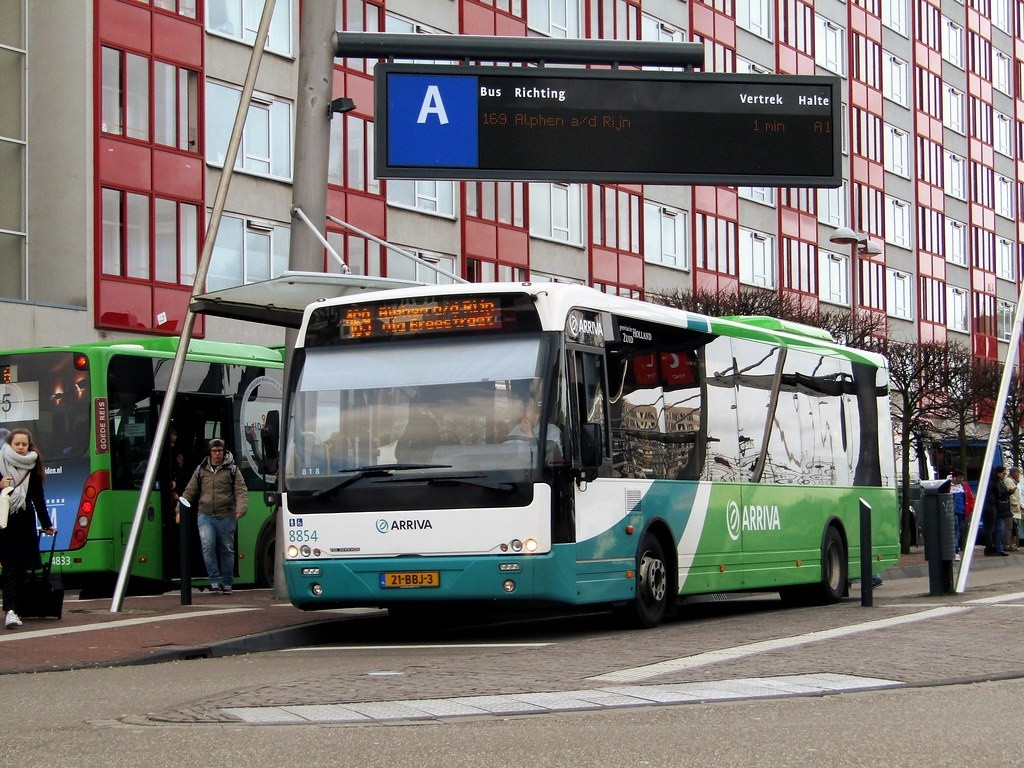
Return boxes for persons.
[159,428,193,562]
[982,466,1024,556]
[64,423,90,456]
[175,439,248,594]
[503,394,562,459]
[0,428,55,626]
[949,469,975,561]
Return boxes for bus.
[272,282,905,625]
[0,338,284,598]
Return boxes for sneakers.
[211,582,221,593]
[5,610,23,628]
[222,584,233,594]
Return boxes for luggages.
[19,529,65,619]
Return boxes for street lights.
[829,226,883,349]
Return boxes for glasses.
[210,449,223,453]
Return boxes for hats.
[209,439,224,448]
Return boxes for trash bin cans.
[918,476,959,600]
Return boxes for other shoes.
[955,553,961,561]
[983,548,1009,556]
[1003,544,1020,551]
